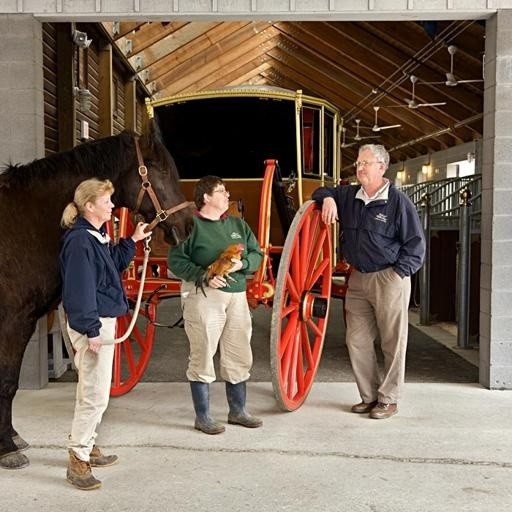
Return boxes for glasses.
[353,161,381,167]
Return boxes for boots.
[190,381,225,434]
[226,381,263,428]
[67,445,118,490]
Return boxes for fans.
[340,46,483,150]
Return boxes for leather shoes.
[352,399,397,419]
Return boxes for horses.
[0,117,193,470]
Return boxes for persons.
[310,143,429,421]
[56,177,154,489]
[165,175,265,437]
